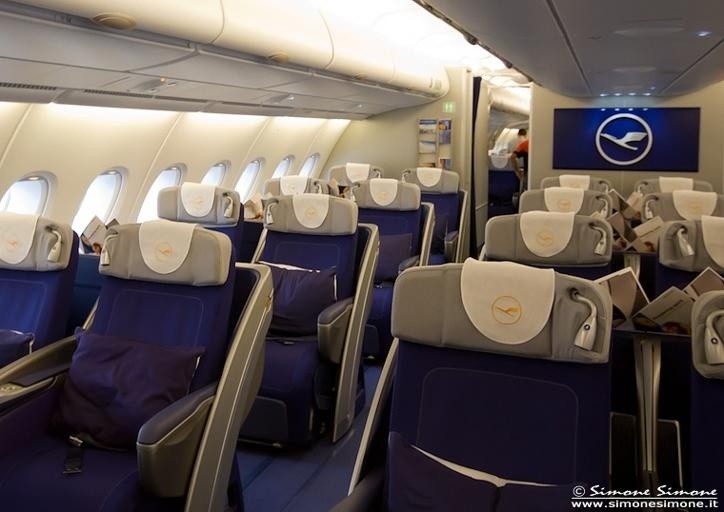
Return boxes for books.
[594,187,724,332]
[105,217,120,230]
[79,216,107,254]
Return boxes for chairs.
[0,161,723,507]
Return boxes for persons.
[511,139,529,191]
[510,128,528,190]
